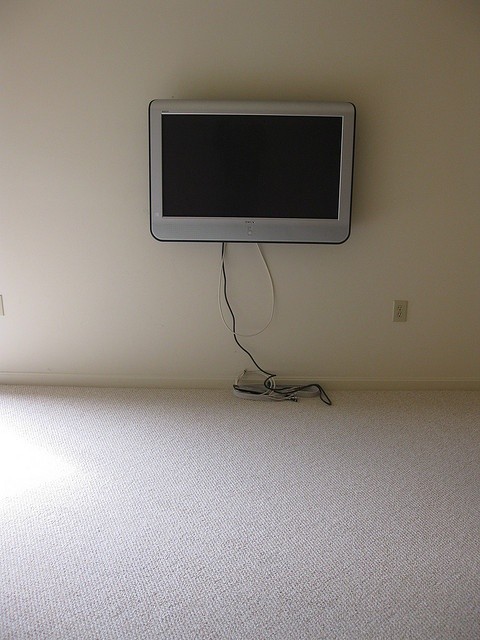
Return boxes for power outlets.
[393,299,408,322]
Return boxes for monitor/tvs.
[146,97,356,247]
